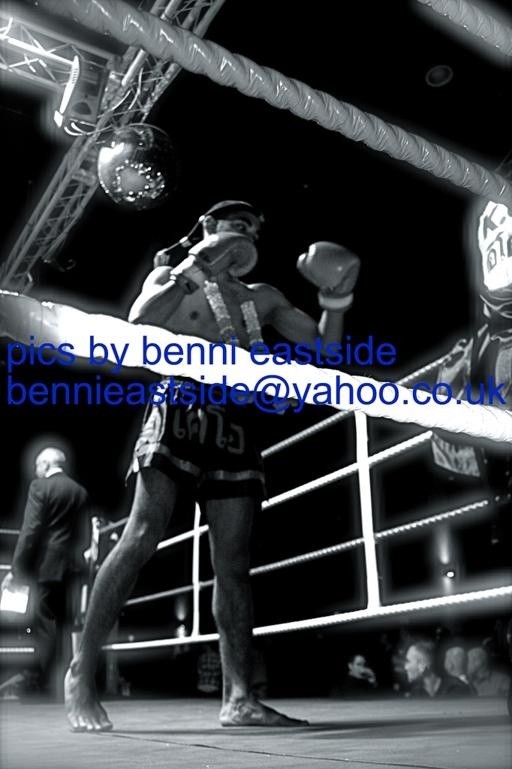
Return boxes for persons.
[3,447,88,703]
[63,198,363,735]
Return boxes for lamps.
[60,54,175,212]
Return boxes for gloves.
[296,242,360,311]
[171,232,260,294]
